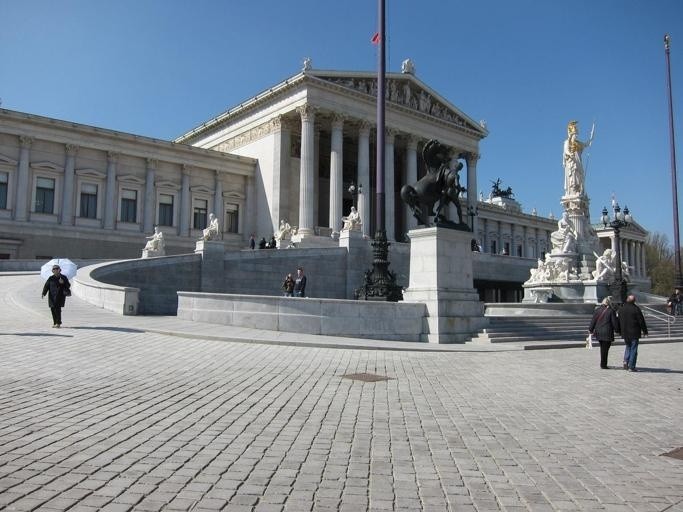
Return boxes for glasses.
[53,267,59,270]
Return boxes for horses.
[400,139,451,228]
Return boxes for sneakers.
[623,361,628,370]
[628,368,638,372]
[52,324,60,328]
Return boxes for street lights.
[600,192,633,307]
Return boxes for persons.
[592,248,631,282]
[342,206,361,231]
[283,273,295,297]
[588,295,620,369]
[293,267,307,297]
[269,236,276,248]
[258,237,266,249]
[667,292,683,315]
[526,254,578,280]
[199,213,219,240]
[275,220,291,240]
[550,210,575,253]
[142,225,164,250]
[248,235,254,249]
[433,162,468,224]
[562,119,594,197]
[41,264,71,328]
[617,295,648,371]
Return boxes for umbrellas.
[41,258,77,280]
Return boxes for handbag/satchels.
[63,288,72,296]
